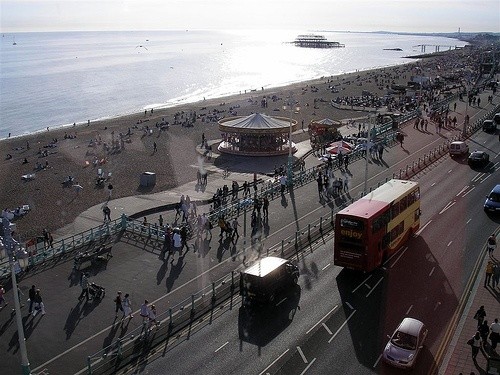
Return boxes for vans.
[449,141,469,159]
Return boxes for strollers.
[88,282,105,296]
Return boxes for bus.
[333,178,422,274]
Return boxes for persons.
[77,272,92,301]
[232,217,242,237]
[491,264,500,289]
[103,206,111,222]
[148,305,160,329]
[42,228,49,248]
[172,228,183,258]
[174,202,181,217]
[246,181,251,195]
[399,134,404,147]
[343,176,350,193]
[470,330,483,361]
[186,194,190,207]
[157,214,163,226]
[242,179,247,195]
[344,154,350,169]
[77,242,107,261]
[324,175,330,189]
[341,178,344,190]
[17,243,29,273]
[478,320,490,349]
[333,175,339,193]
[412,116,420,130]
[16,284,25,309]
[483,260,493,289]
[338,151,343,167]
[278,173,288,197]
[231,182,236,200]
[196,168,202,184]
[372,141,377,160]
[80,272,85,289]
[377,139,389,160]
[195,214,203,237]
[473,305,487,330]
[164,228,173,254]
[180,193,185,204]
[113,290,125,318]
[489,319,500,350]
[218,214,227,238]
[142,216,148,226]
[257,196,263,217]
[107,182,113,197]
[200,213,209,235]
[47,232,54,249]
[316,173,323,192]
[361,140,367,157]
[338,178,342,192]
[203,171,209,185]
[208,145,213,156]
[263,195,270,217]
[140,299,149,325]
[202,218,214,239]
[180,226,189,253]
[250,193,258,217]
[327,153,333,170]
[28,285,36,314]
[235,180,239,199]
[487,233,498,258]
[424,119,428,131]
[369,139,373,158]
[32,289,46,318]
[122,294,134,321]
[226,221,232,236]
[420,117,425,130]
[91,280,105,292]
[0,285,8,309]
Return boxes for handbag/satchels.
[466,338,474,345]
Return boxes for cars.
[467,149,489,166]
[483,184,500,214]
[382,317,429,370]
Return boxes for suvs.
[483,119,497,131]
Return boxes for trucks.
[239,256,300,311]
